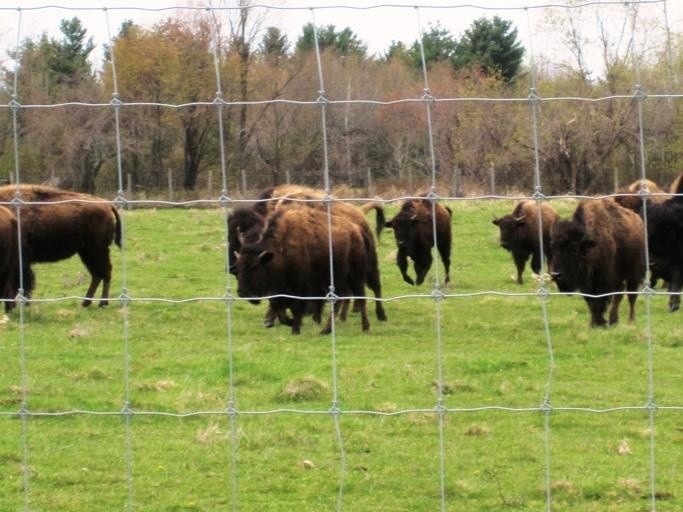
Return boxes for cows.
[546,170,683,328]
[0,183,121,324]
[226,181,386,336]
[386,191,454,287]
[490,200,561,285]
